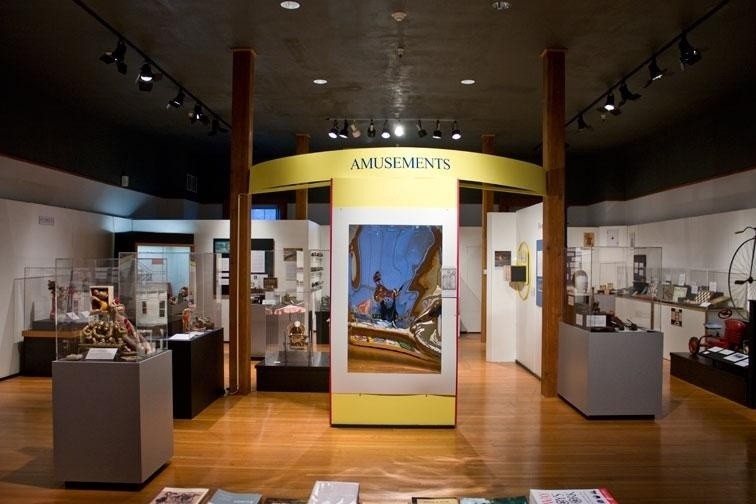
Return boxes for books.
[412,497,460,504]
[308,480,360,504]
[528,488,618,504]
[205,488,263,504]
[150,486,212,504]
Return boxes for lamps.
[326,118,461,140]
[565,2,729,131]
[73,0,231,134]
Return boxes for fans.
[728,237,756,321]
